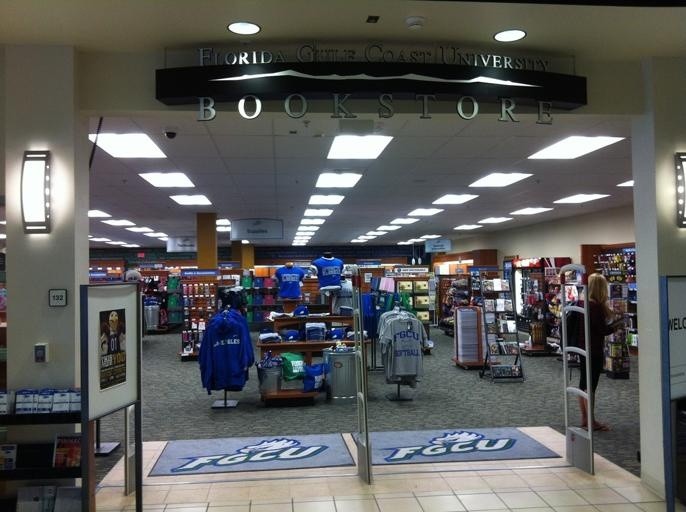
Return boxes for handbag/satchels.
[558,301,579,354]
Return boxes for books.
[482,278,522,377]
[604,285,630,373]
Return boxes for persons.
[310,250,346,293]
[121,262,143,281]
[271,259,308,297]
[100,311,126,355]
[577,272,624,430]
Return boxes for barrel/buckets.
[322,346,361,406]
[144,305,160,329]
[257,366,280,393]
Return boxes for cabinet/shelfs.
[544,276,637,380]
[177,279,237,363]
[436,273,502,338]
[142,269,295,337]
[321,266,430,355]
[0,411,82,512]
[253,315,356,402]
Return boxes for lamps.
[674,152,686,228]
[494,28,526,43]
[226,22,262,36]
[19,150,50,234]
